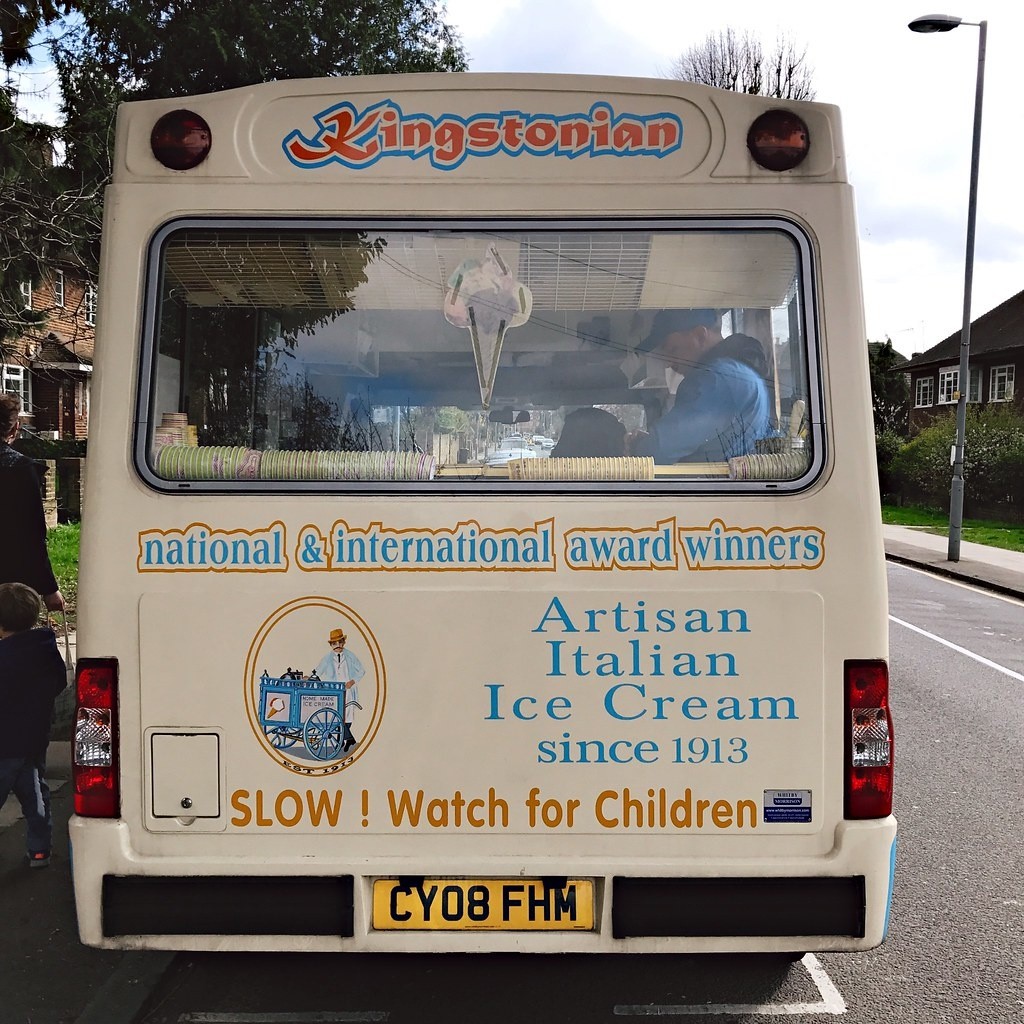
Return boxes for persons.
[0,392,65,871]
[552,309,771,465]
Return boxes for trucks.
[66,78,896,961]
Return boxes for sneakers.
[28,849,53,869]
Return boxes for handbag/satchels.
[45,609,77,742]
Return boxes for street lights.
[911,13,988,563]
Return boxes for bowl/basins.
[509,456,655,479]
[153,412,436,480]
[728,452,808,481]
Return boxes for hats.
[635,310,719,356]
